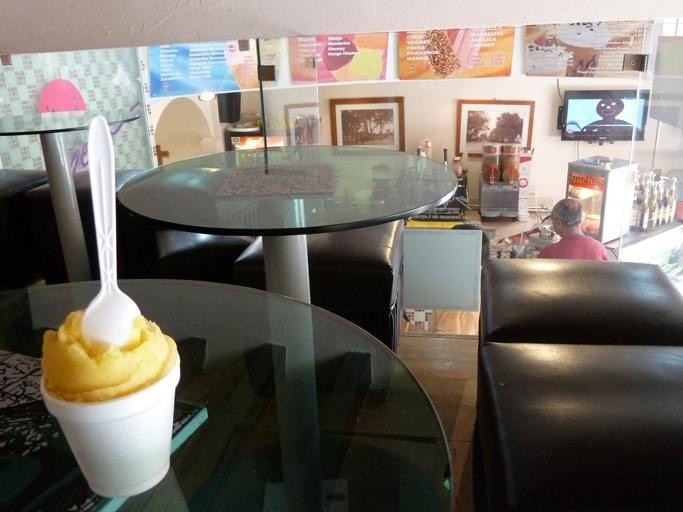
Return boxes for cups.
[38,347,183,500]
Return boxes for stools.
[0,167,405,357]
[482,258,683,346]
[482,341,683,512]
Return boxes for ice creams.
[526,37,568,72]
[560,22,607,46]
[41,307,177,404]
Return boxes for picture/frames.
[455,101,533,163]
[328,96,405,154]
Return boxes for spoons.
[78,113,144,349]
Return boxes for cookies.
[422,30,462,79]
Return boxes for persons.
[536,198,608,261]
[423,224,491,337]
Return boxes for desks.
[0,278,457,510]
[117,144,458,304]
[1,110,143,283]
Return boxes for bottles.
[628,171,678,234]
